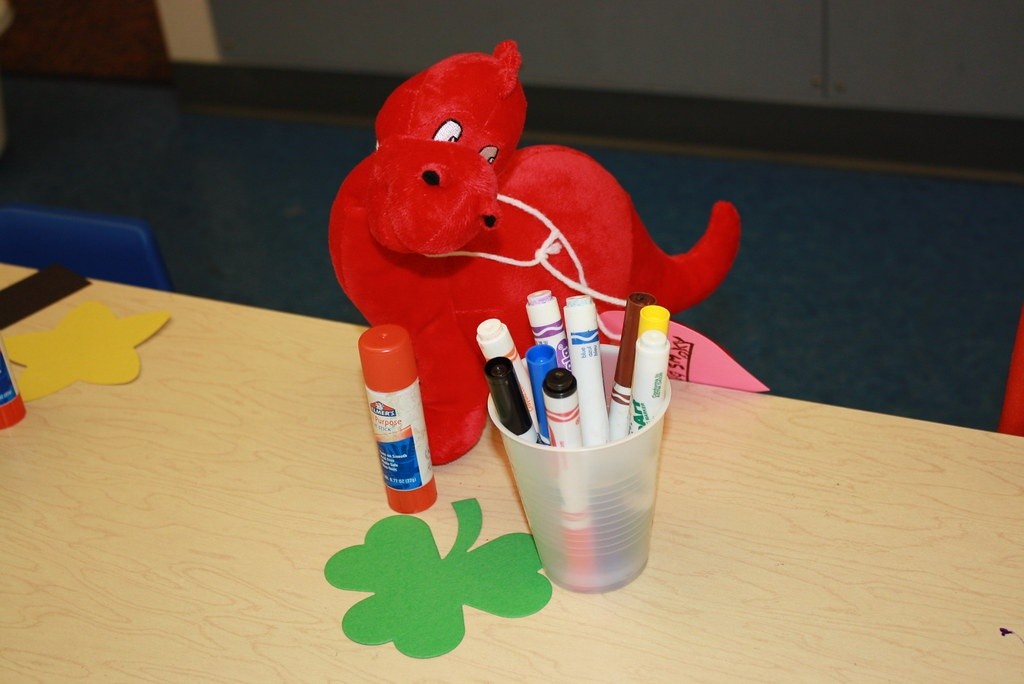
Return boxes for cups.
[487,344,671,593]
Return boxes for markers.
[476,289,671,445]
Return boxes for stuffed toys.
[328,38,744,466]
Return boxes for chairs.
[0,205,174,292]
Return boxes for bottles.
[358,323,438,514]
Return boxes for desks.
[0,261,1024,684]
